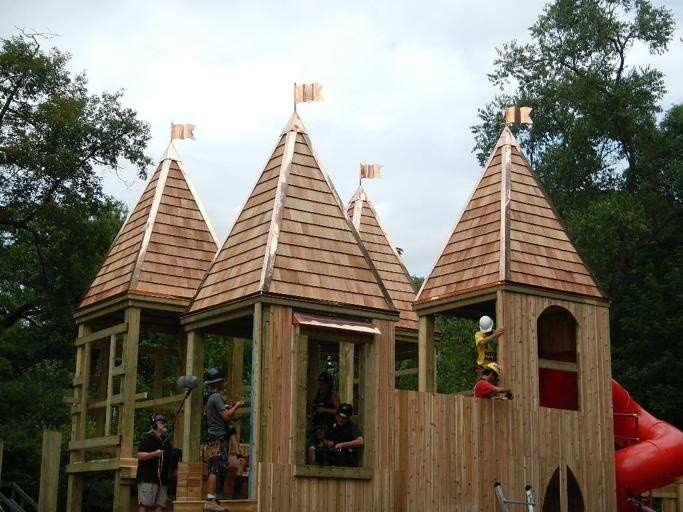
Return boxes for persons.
[304,372,364,468]
[225,423,247,500]
[202,367,245,512]
[475,315,505,370]
[473,362,512,398]
[138,413,173,512]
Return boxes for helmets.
[337,402,353,419]
[204,368,224,384]
[479,315,493,333]
[484,362,500,374]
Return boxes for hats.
[154,415,167,421]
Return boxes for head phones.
[152,414,158,430]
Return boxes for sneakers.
[203,500,229,512]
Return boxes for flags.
[294,84,322,102]
[507,107,533,124]
[172,124,196,140]
[361,165,381,179]
